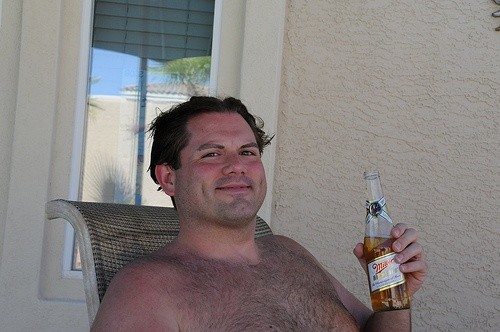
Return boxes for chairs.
[46,198,277,332]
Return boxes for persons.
[87,95,428,331]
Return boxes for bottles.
[362,170,410,312]
